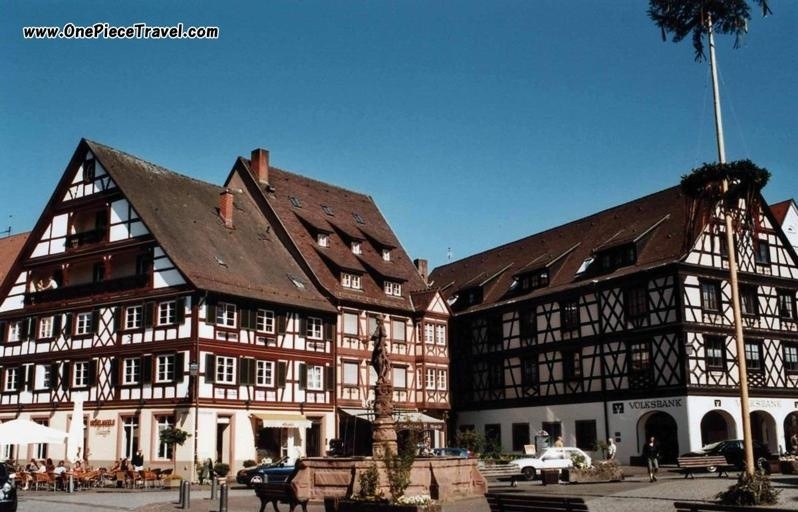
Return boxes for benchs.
[672,455,735,480]
[475,462,592,512]
[255,482,310,511]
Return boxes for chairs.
[9,467,174,493]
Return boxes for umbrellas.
[0,420,65,467]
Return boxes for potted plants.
[214,463,230,485]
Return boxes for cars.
[504,447,592,480]
[678,439,772,473]
[433,447,479,459]
[237,456,300,488]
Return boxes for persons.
[37,275,58,291]
[554,436,564,447]
[196,458,220,485]
[643,436,660,482]
[3,446,144,491]
[607,438,617,460]
